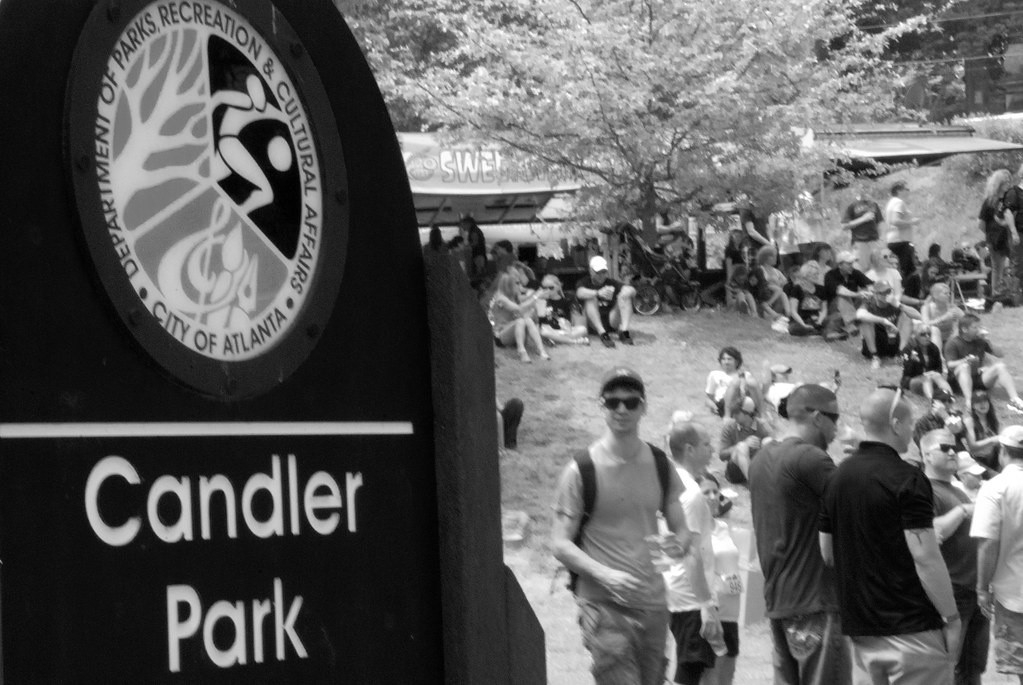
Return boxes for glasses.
[540,285,555,290]
[928,443,958,454]
[805,406,840,423]
[882,255,893,259]
[600,397,644,411]
[920,331,931,337]
[940,398,954,404]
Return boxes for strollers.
[614,219,704,318]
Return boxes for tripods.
[934,267,970,314]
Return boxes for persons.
[705,346,1023,684]
[423,215,590,360]
[723,162,1022,415]
[659,409,741,685]
[552,365,690,685]
[576,255,639,348]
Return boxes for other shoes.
[617,330,633,345]
[600,333,614,348]
[517,349,531,364]
[826,331,849,341]
[574,337,590,345]
[871,357,881,371]
[1006,396,1023,413]
[536,349,550,361]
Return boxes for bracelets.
[532,295,537,300]
[962,504,970,515]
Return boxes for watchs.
[942,614,963,625]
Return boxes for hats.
[971,389,990,404]
[770,364,792,376]
[956,460,986,475]
[836,251,859,263]
[931,388,953,400]
[599,365,644,396]
[874,279,894,293]
[589,255,608,272]
[999,424,1023,448]
